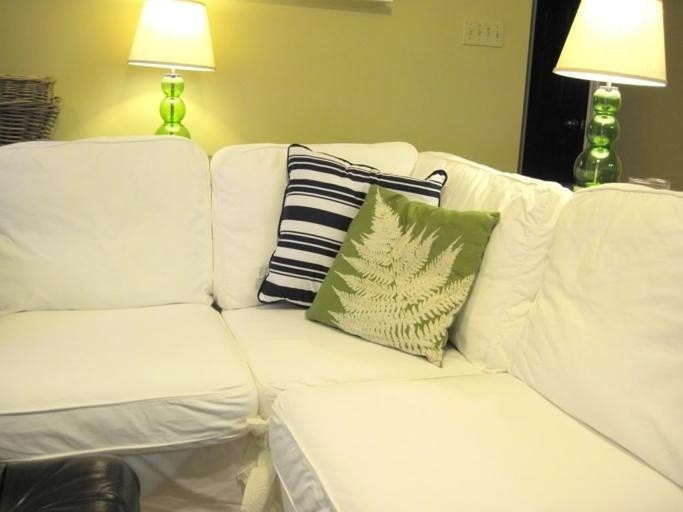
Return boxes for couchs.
[3,140,681,510]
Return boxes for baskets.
[0,72,63,145]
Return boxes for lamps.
[550,0,668,188]
[127,1,216,139]
